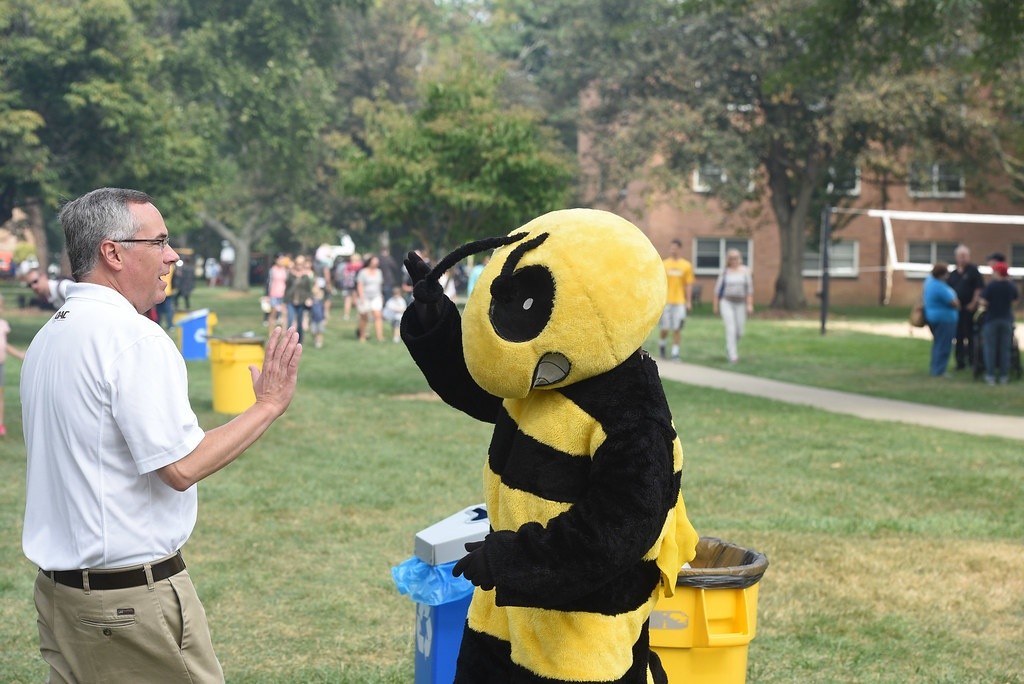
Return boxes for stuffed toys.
[400,207,699,684]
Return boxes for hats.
[990,262,1009,278]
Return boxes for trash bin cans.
[203,335,265,413]
[172,310,218,353]
[646,537,769,684]
[416,503,488,684]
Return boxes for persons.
[0,296,26,435]
[260,251,332,348]
[713,250,753,360]
[467,255,490,294]
[923,262,960,376]
[145,258,195,329]
[438,272,455,299]
[22,187,302,684]
[206,240,236,287]
[25,268,74,309]
[336,246,425,343]
[660,239,694,356]
[948,245,1019,385]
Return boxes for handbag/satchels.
[911,305,925,329]
[717,267,727,300]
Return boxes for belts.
[41,552,185,590]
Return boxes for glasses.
[113,235,170,249]
[26,275,41,288]
